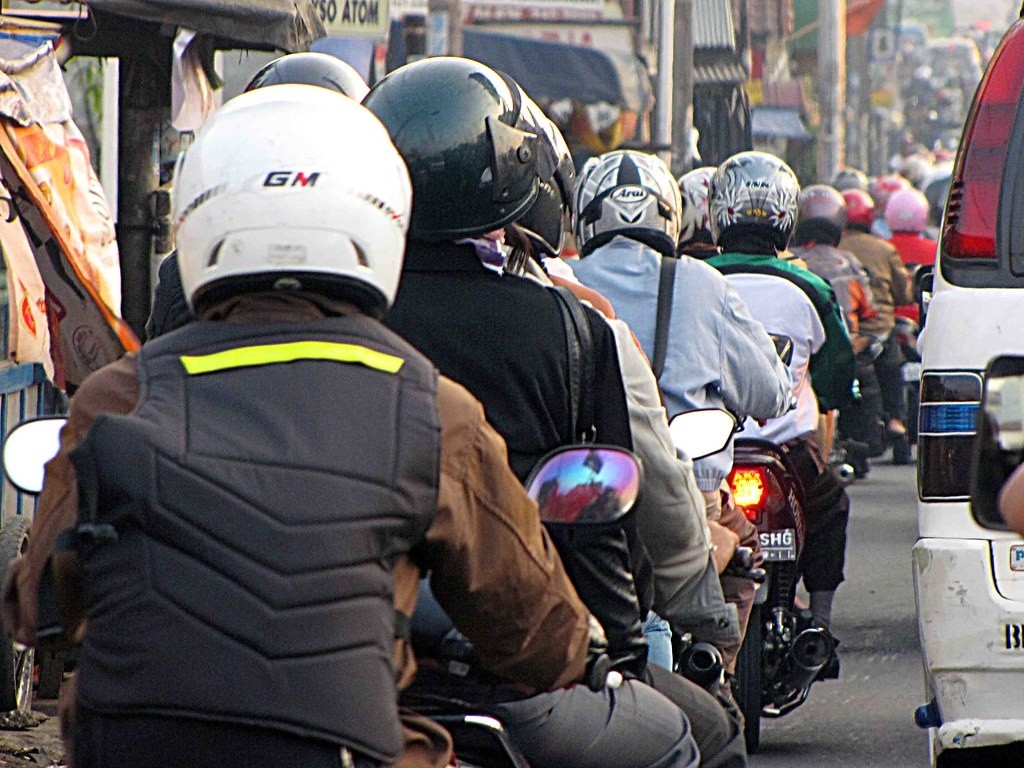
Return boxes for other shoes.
[713,679,746,728]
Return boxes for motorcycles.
[1,260,933,767]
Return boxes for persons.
[0,85,591,768]
[142,53,1024,768]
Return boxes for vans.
[902,7,1023,768]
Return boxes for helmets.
[793,184,847,246]
[841,188,875,233]
[707,152,800,250]
[676,167,722,248]
[243,51,371,104]
[513,120,577,256]
[360,57,559,243]
[168,83,412,313]
[833,168,867,192]
[884,188,927,235]
[574,148,683,258]
